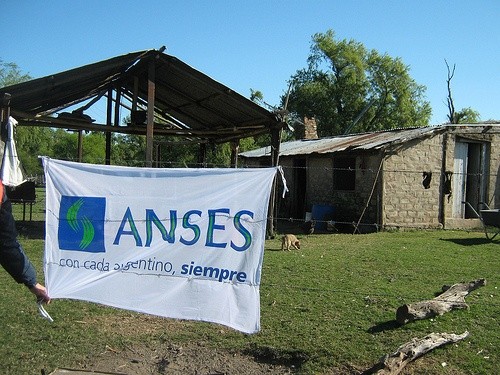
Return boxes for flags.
[37,154,290,335]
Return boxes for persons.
[0,179,51,306]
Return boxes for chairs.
[6,180,36,224]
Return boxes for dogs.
[278,234,301,252]
